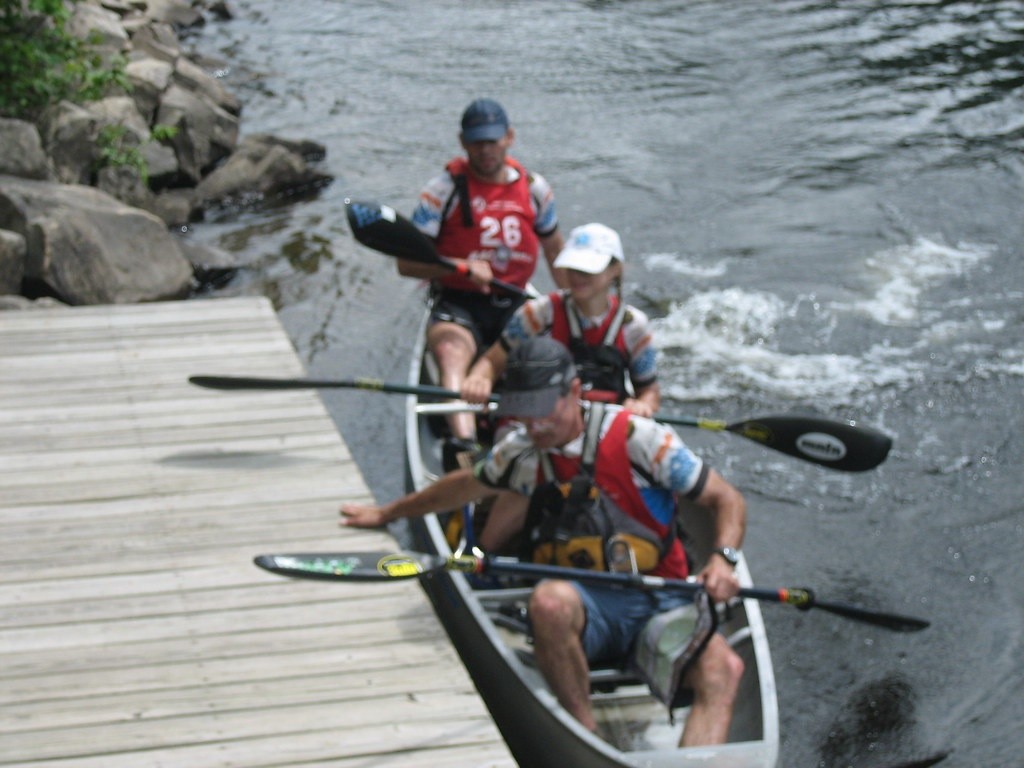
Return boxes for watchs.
[714,546,739,566]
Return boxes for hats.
[461,97,509,140]
[553,223,625,274]
[492,336,574,417]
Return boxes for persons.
[339,336,748,748]
[395,97,570,473]
[461,221,660,575]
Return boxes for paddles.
[253,550,934,634]
[345,201,537,299]
[188,356,898,475]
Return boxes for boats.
[402,276,784,767]
